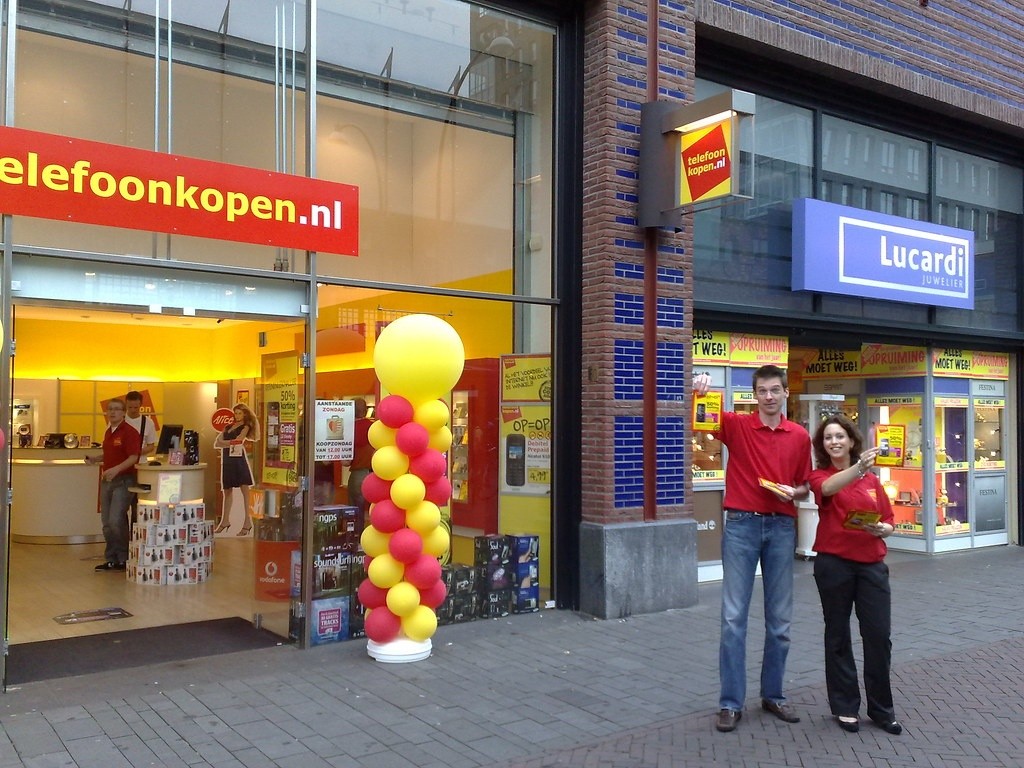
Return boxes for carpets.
[5,617,291,686]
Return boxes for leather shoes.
[717,706,748,731]
[761,698,800,722]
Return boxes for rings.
[866,458,869,462]
[868,453,871,456]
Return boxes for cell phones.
[506,434,526,486]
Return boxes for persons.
[693,366,903,736]
[84,391,159,572]
[214,403,260,536]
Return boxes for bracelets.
[855,459,865,480]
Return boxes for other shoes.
[95,561,126,572]
[833,712,865,733]
[873,713,902,735]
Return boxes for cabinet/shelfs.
[890,467,964,534]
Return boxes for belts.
[724,508,797,518]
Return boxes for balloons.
[0,318,5,452]
[357,313,465,644]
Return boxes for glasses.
[108,406,127,415]
[758,385,784,396]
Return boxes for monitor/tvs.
[156,424,183,454]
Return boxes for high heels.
[237,523,253,538]
[213,521,233,534]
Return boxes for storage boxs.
[290,505,539,645]
[126,503,215,586]
